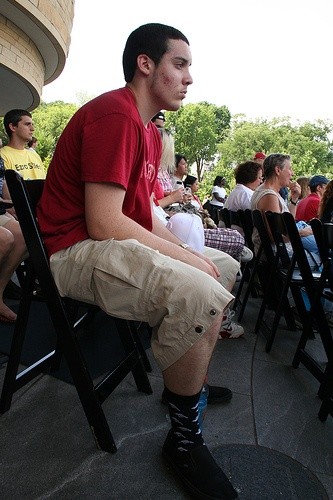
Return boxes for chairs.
[0,169,333,457]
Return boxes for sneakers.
[217,308,244,339]
[162,382,233,405]
[161,428,239,500]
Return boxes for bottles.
[175,181,192,203]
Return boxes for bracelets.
[178,242,189,250]
[290,198,297,207]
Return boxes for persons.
[0,109,46,322]
[151,111,333,339]
[37,23,240,500]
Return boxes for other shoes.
[295,318,319,332]
[324,311,333,328]
[241,245,253,262]
[235,268,242,282]
[0,303,17,323]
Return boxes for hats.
[310,175,329,187]
[152,112,165,120]
[255,153,266,159]
